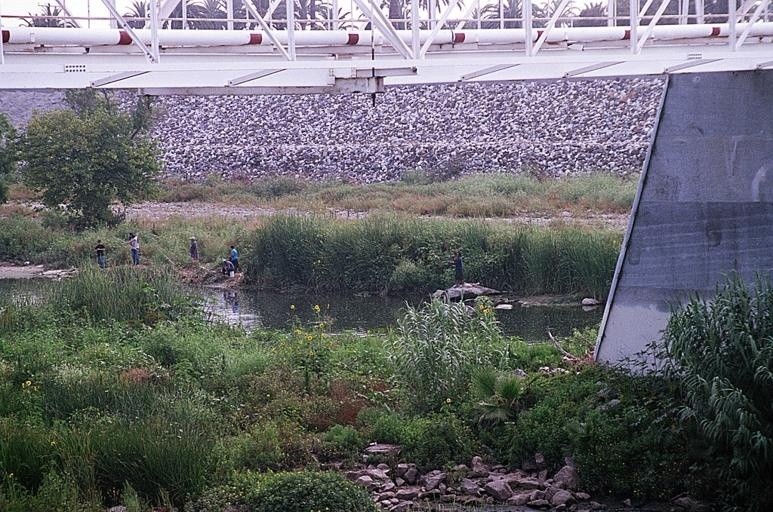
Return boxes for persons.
[184,236,199,261]
[120,232,139,265]
[228,246,238,273]
[93,239,106,268]
[448,250,464,288]
[221,259,234,277]
